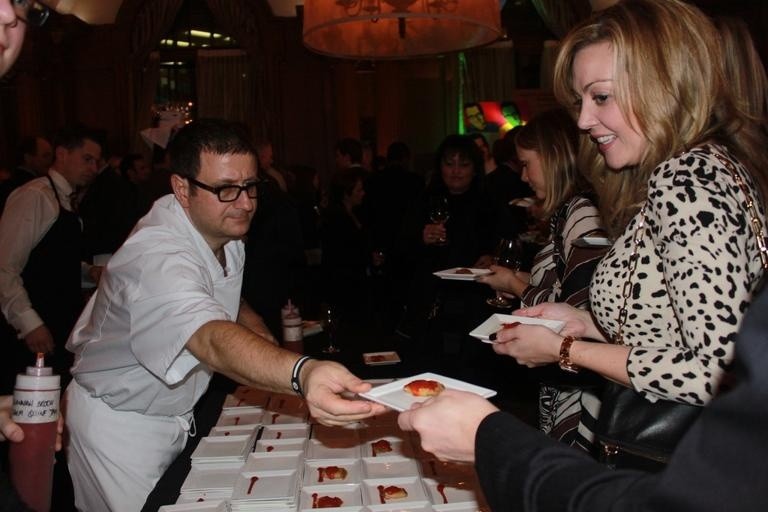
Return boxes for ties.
[70,192,80,215]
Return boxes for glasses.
[192,177,265,203]
[10,1,53,29]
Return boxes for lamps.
[301,2,502,63]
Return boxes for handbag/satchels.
[591,378,706,474]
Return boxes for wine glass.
[486,238,523,310]
[427,195,451,247]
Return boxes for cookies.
[321,468,347,479]
[316,496,344,509]
[372,440,392,457]
[404,380,446,396]
[384,487,407,500]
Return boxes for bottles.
[7,350,63,512]
[279,296,305,356]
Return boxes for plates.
[359,371,499,418]
[468,312,567,346]
[360,350,401,368]
[157,407,474,512]
[429,265,494,282]
